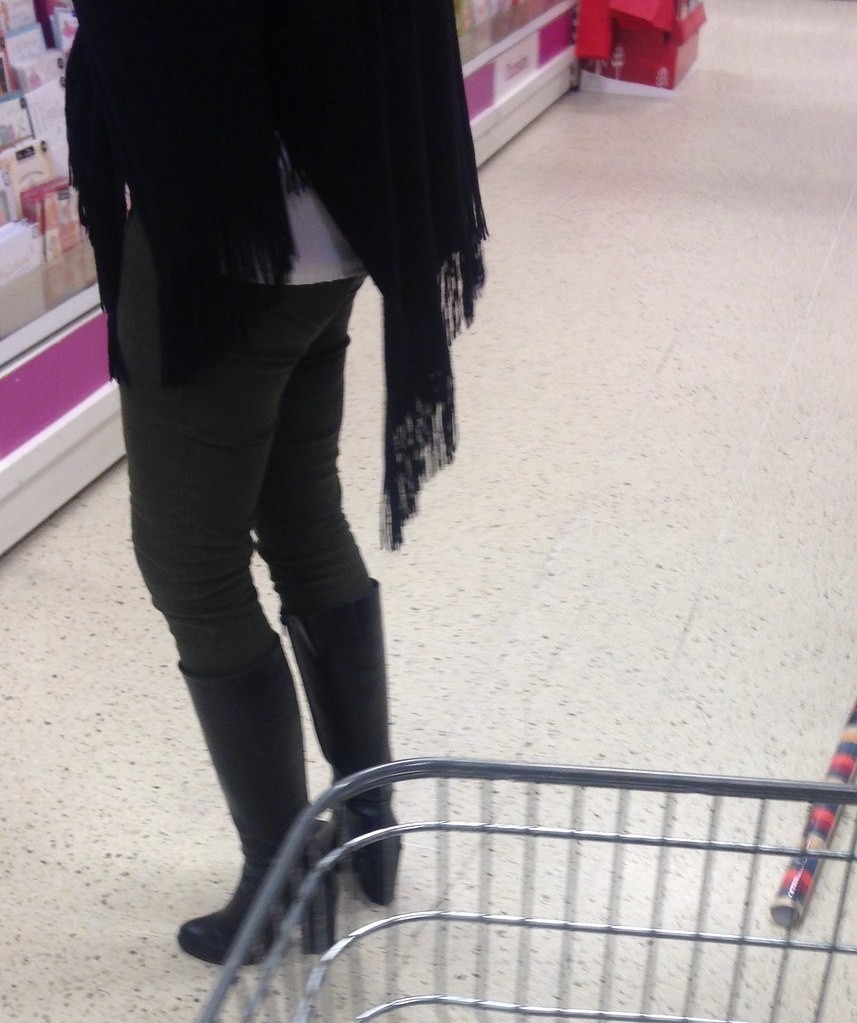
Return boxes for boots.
[281,577,401,904]
[176,633,339,965]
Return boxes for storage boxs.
[0,238,98,341]
[576,0,706,96]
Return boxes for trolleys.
[194,753,856,1023]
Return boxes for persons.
[64,0,492,965]
